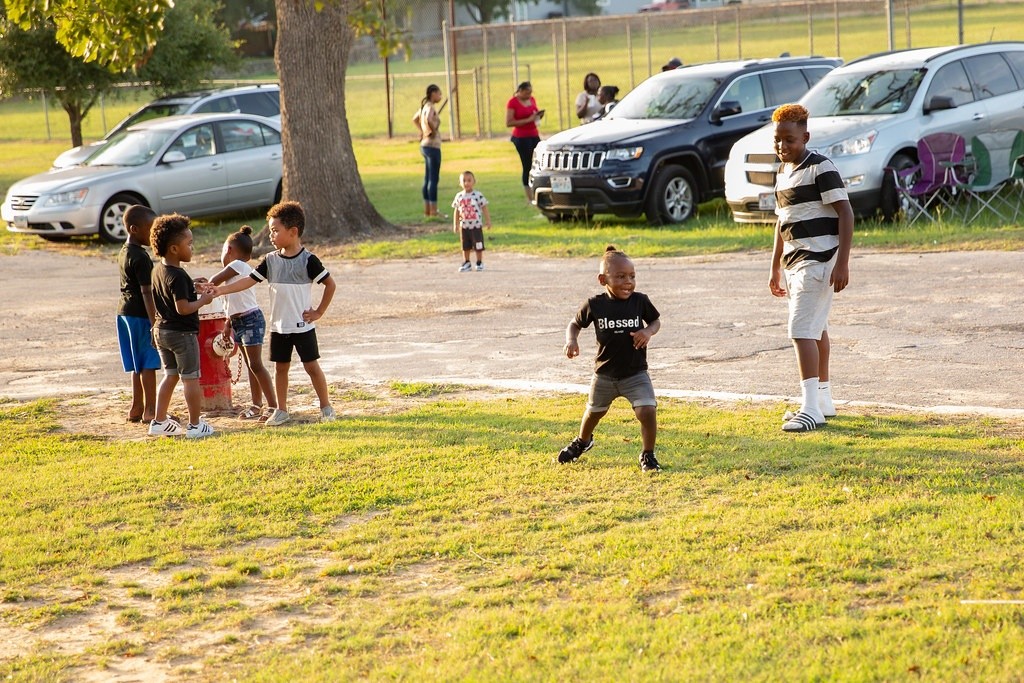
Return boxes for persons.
[663,59,682,72]
[451,171,491,273]
[506,81,544,203]
[199,225,277,424]
[413,84,448,218]
[146,213,215,438]
[116,204,182,424]
[208,202,337,425]
[557,245,664,473]
[768,104,855,432]
[575,73,619,124]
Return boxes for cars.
[722,41,1024,224]
[1,113,282,243]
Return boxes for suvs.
[50,81,281,173]
[528,52,845,227]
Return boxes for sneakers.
[639,450,660,473]
[558,434,593,465]
[321,405,335,423]
[185,419,215,438]
[459,261,471,272]
[145,418,186,437]
[265,409,290,426]
[475,263,483,272]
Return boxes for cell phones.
[537,110,545,115]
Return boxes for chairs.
[192,129,214,158]
[885,128,1024,227]
[158,134,185,161]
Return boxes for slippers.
[783,412,828,430]
[785,408,838,421]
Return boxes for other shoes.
[143,415,181,424]
[127,414,142,421]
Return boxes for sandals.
[261,405,276,418]
[237,402,261,420]
